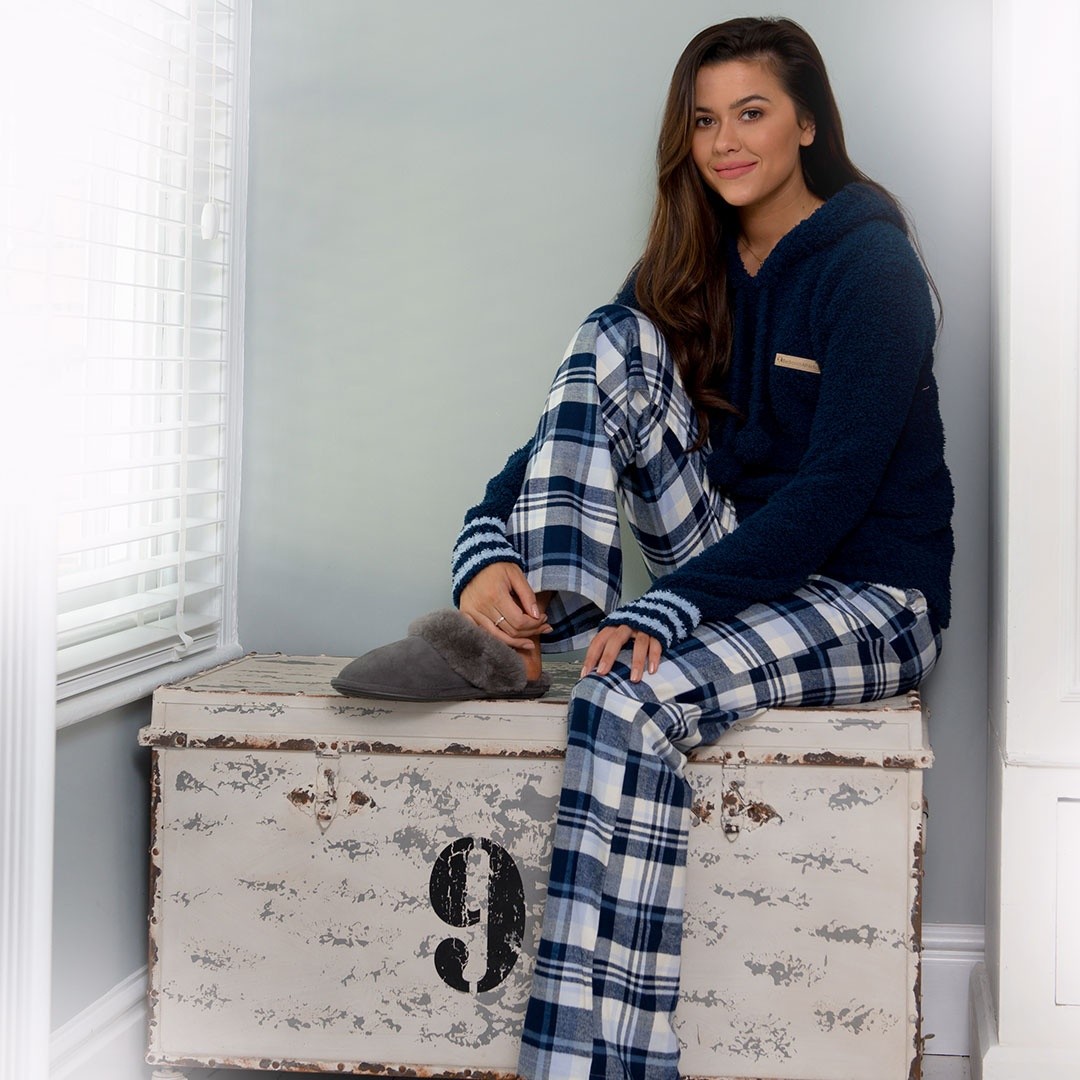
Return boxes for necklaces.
[738,233,763,267]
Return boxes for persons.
[327,15,956,1080]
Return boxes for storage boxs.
[135,652,930,1080]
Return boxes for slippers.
[326,606,552,701]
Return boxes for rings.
[494,617,504,626]
